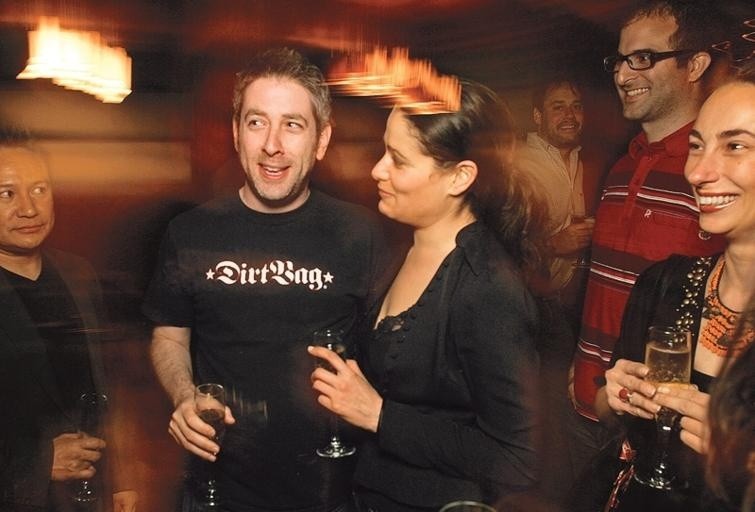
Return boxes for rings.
[619,386,635,403]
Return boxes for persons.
[136,45,412,512]
[508,74,602,512]
[589,57,754,510]
[304,73,551,512]
[0,124,144,511]
[702,341,754,510]
[567,0,725,511]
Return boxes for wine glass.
[69,394,108,504]
[193,384,228,508]
[312,330,357,460]
[633,323,691,492]
[569,193,591,270]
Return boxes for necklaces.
[701,257,754,359]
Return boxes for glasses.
[602,49,692,73]
